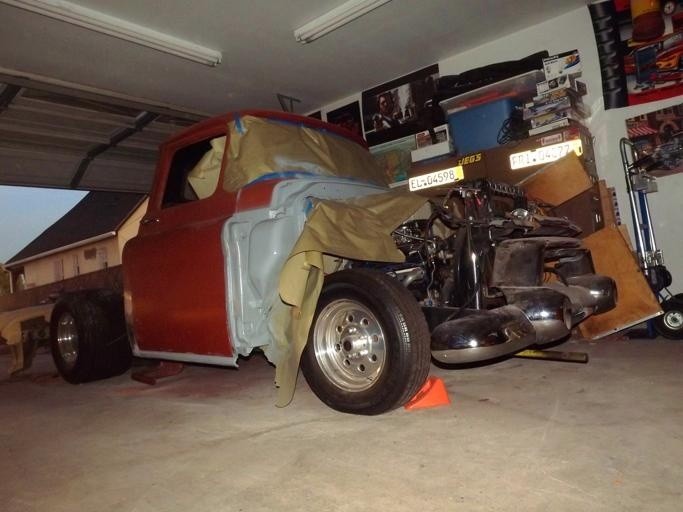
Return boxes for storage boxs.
[407,48,589,167]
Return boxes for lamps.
[1,0,222,68]
[294,0,389,46]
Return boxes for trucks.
[0,107,617,417]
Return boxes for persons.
[373,93,399,132]
